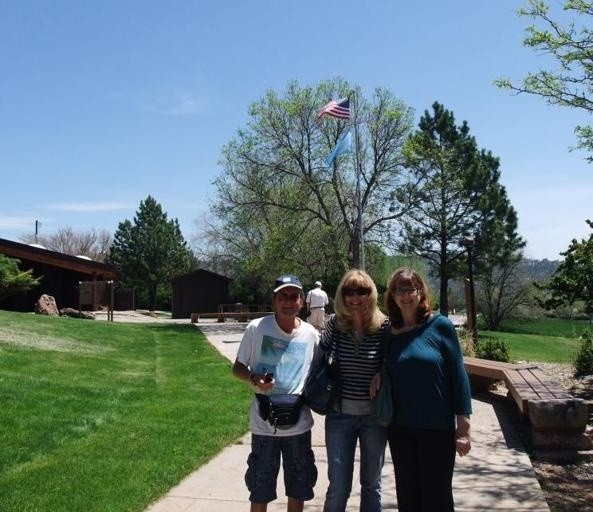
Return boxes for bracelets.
[248,372,255,380]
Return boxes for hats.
[314,281,322,287]
[273,275,303,293]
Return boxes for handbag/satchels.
[304,351,341,415]
[253,394,304,425]
[371,371,395,427]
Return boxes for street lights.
[34,219,42,243]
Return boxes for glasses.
[341,288,367,295]
[392,286,417,295]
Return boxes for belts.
[311,307,324,308]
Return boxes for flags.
[318,96,350,120]
[326,128,353,169]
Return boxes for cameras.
[306,311,311,317]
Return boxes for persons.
[232,274,321,512]
[313,268,391,512]
[378,267,473,512]
[305,281,329,333]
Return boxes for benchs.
[463,358,592,452]
[190,312,265,323]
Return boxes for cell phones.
[265,372,273,383]
